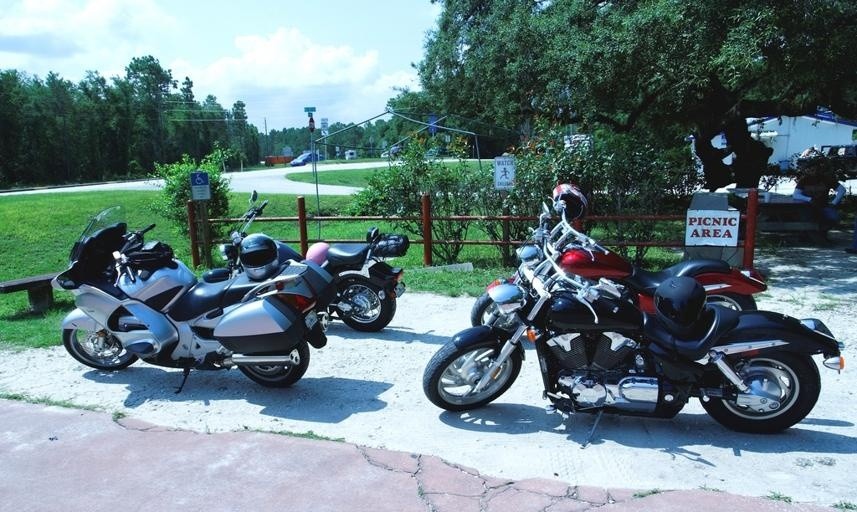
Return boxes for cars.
[389,147,407,162]
[380,151,389,159]
[289,153,327,166]
[422,147,452,158]
[515,134,592,160]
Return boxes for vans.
[345,150,356,160]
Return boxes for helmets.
[239,233,280,281]
[651,275,707,335]
[552,182,587,222]
[305,241,329,268]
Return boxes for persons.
[501,146,515,158]
[793,165,846,239]
[844,216,857,255]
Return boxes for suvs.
[788,143,856,181]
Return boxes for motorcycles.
[50,205,337,394]
[216,189,410,332]
[422,201,843,450]
[469,183,767,351]
